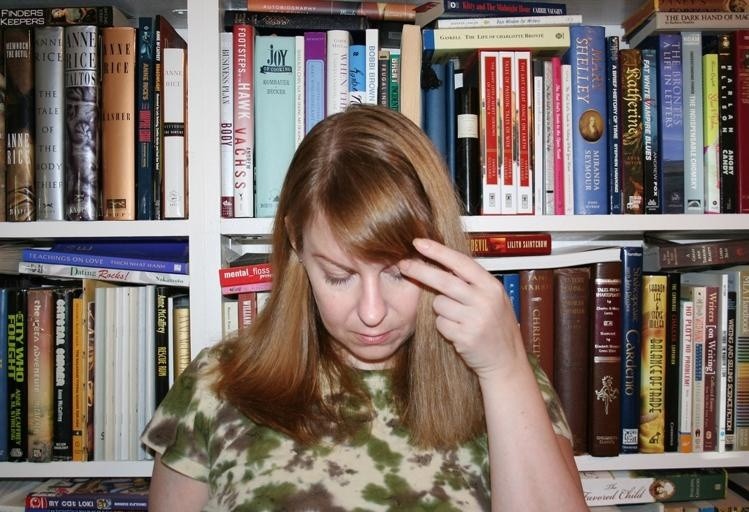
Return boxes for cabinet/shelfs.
[1,1,748,511]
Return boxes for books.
[451,228,749,455]
[1,1,748,222]
[579,468,727,507]
[1,234,272,463]
[22,479,153,511]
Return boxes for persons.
[139,101,595,512]
